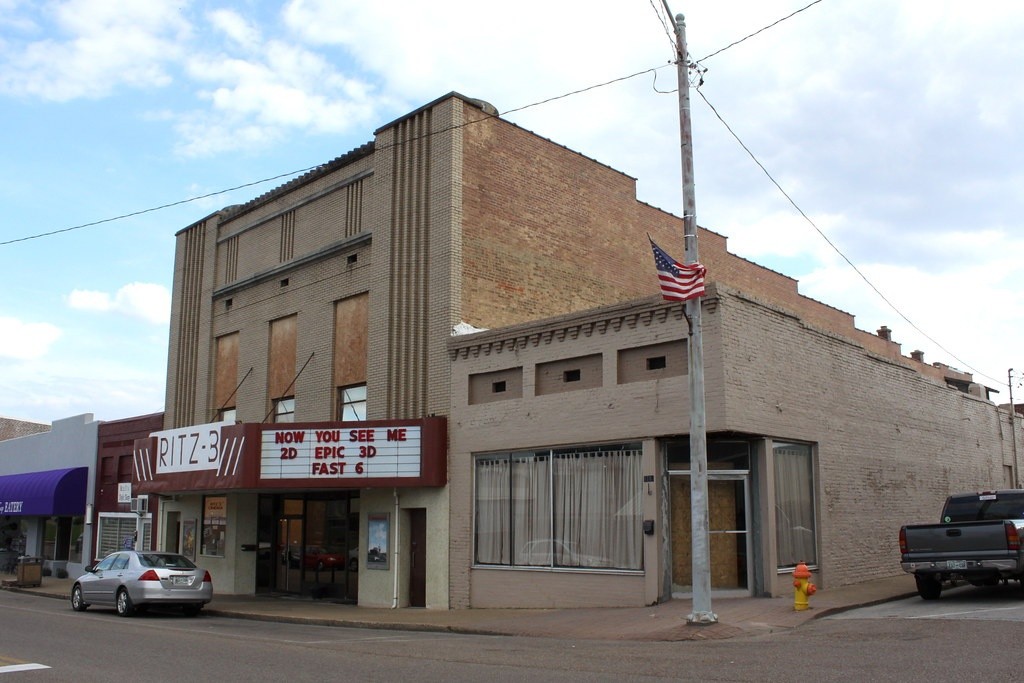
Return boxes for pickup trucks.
[899,488,1024,600]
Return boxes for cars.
[70,550,213,619]
[349,546,359,572]
[281,544,344,573]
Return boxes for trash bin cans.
[19,556,43,589]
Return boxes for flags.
[650,239,707,304]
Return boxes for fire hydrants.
[791,561,817,612]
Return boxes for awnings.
[0,466,89,517]
